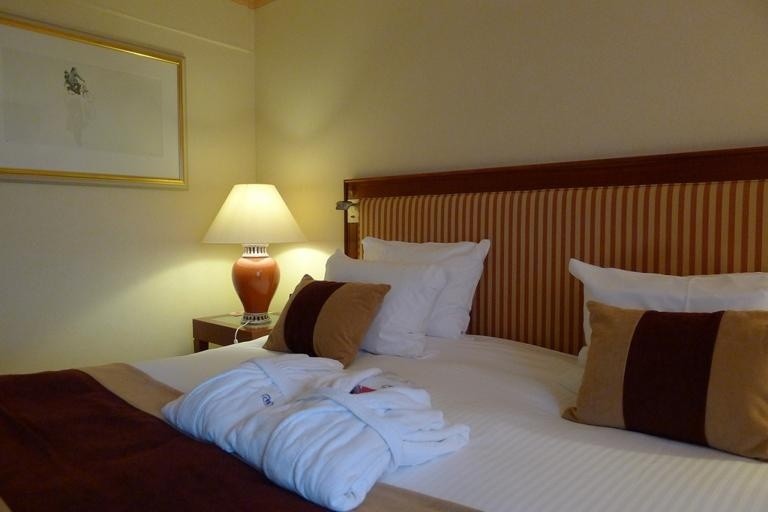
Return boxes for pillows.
[323,248,450,358]
[359,235,492,341]
[563,300,768,464]
[262,273,392,370]
[558,255,768,400]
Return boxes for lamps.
[200,182,311,327]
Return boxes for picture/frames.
[1,10,192,194]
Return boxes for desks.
[190,310,283,353]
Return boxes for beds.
[0,332,768,511]
